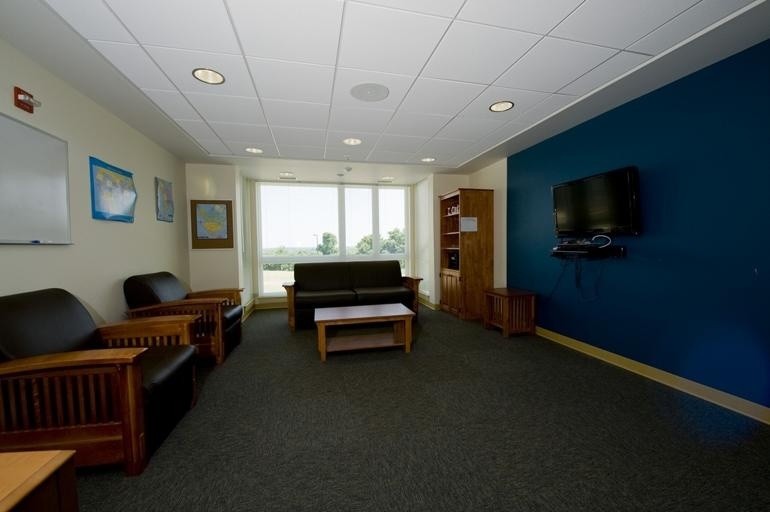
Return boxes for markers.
[31,240,45,243]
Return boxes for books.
[446,204,460,216]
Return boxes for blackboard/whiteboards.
[0,111,73,245]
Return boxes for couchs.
[282,261,423,332]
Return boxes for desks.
[0,449,79,511]
[484,288,536,339]
[314,303,416,362]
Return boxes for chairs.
[123,271,244,366]
[0,287,203,476]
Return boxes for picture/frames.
[190,199,233,249]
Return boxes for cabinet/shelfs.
[437,187,494,321]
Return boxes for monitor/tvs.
[550,167,642,238]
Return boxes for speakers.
[613,245,627,257]
